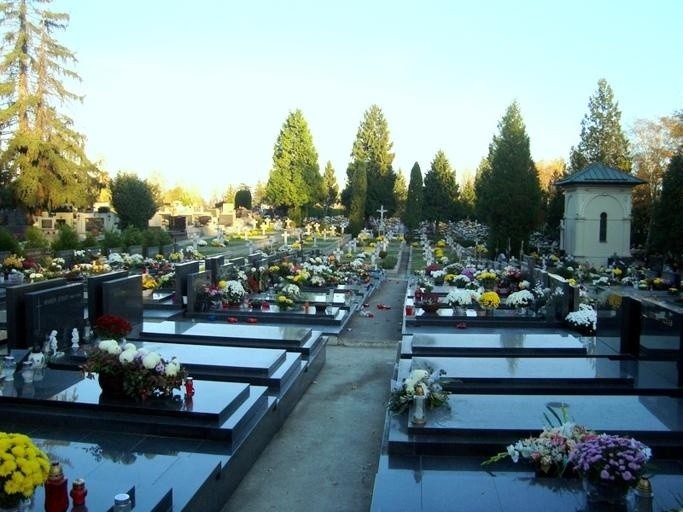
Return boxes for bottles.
[2,355,17,382]
[21,361,34,383]
[27,344,45,382]
[113,494,132,512]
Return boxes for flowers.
[475,402,654,488]
[196,246,368,323]
[1,431,53,502]
[408,252,539,313]
[78,313,186,392]
